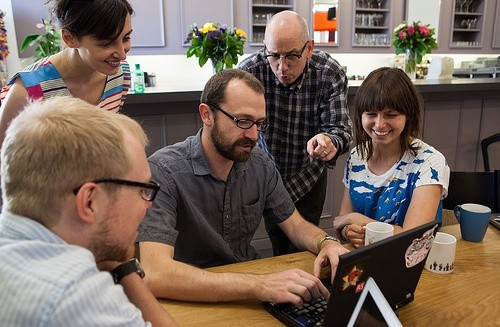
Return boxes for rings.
[324,150,328,155]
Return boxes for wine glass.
[354,0,389,45]
[456,0,476,29]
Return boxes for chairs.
[442,133,500,213]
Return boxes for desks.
[155,222,500,327]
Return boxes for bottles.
[133,64,145,94]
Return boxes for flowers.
[183,23,247,72]
[393,20,438,63]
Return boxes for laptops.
[262,218,442,327]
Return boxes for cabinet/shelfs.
[12,0,500,59]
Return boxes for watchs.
[317,234,341,254]
[111,258,145,285]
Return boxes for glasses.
[73,179,160,201]
[206,102,270,132]
[262,40,310,63]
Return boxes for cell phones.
[490,216,500,229]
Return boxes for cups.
[454,203,492,242]
[253,0,287,43]
[424,231,457,274]
[363,221,395,246]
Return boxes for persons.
[139,69,351,309]
[0,0,136,145]
[0,94,180,327]
[333,67,451,249]
[236,10,357,257]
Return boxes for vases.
[405,48,416,81]
[212,59,226,75]
